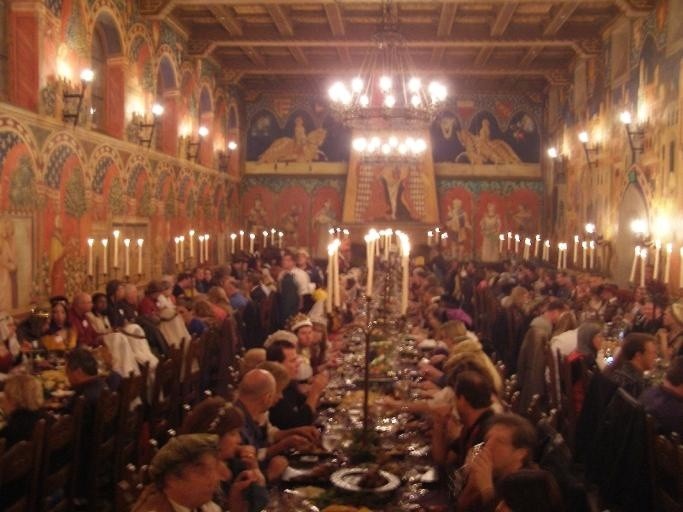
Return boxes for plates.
[328,463,401,494]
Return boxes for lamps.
[219,142,237,169]
[579,132,598,169]
[546,147,566,174]
[352,134,426,163]
[64,69,94,125]
[327,13,447,131]
[188,127,209,159]
[141,104,163,148]
[620,111,644,159]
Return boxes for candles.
[323,227,411,317]
[495,224,595,270]
[627,238,683,290]
[426,228,450,248]
[83,230,144,279]
[230,228,284,254]
[172,230,209,266]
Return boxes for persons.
[446,198,472,259]
[247,197,268,232]
[373,243,683,512]
[279,204,300,248]
[480,203,502,261]
[313,200,338,259]
[1,243,367,512]
[512,202,531,232]
